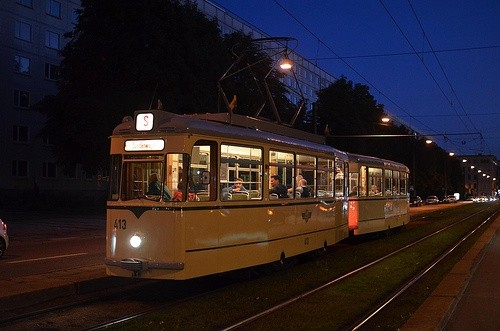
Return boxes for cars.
[467,194,500,202]
[447,195,457,203]
[426,196,440,204]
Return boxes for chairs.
[199,188,325,200]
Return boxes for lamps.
[279,52,292,69]
[381,114,390,122]
[425,138,432,143]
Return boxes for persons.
[288,179,310,198]
[188,192,201,201]
[229,178,249,194]
[369,185,382,196]
[172,183,183,201]
[145,173,171,202]
[296,175,314,198]
[350,186,360,195]
[269,175,289,198]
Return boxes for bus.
[96,37,410,288]
